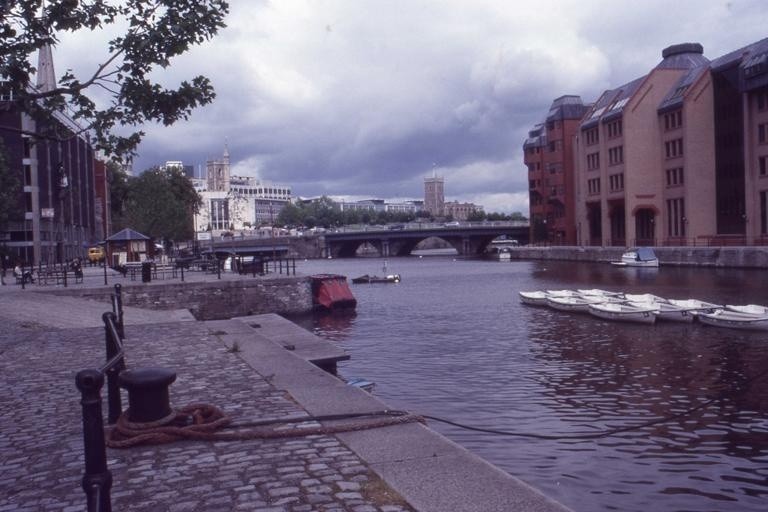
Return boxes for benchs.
[12,264,84,285]
[125,262,177,278]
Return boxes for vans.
[87,247,104,262]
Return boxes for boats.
[350,260,402,284]
[517,288,767,332]
[489,246,511,260]
[621,252,659,267]
[610,261,627,266]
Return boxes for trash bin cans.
[142,263,151,282]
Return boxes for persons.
[14,262,37,284]
[69,257,83,283]
[0,254,8,286]
